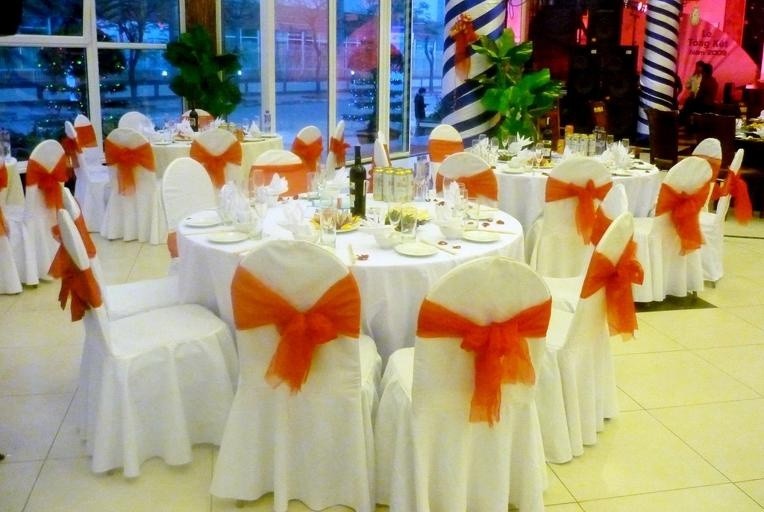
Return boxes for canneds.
[565,133,596,157]
[219,122,237,132]
[372,166,414,203]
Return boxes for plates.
[208,230,248,242]
[184,210,222,228]
[463,230,501,243]
[297,192,323,199]
[393,241,440,256]
[466,208,497,220]
[261,133,279,138]
[151,117,264,146]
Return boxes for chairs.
[0,107,764,512]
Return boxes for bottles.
[349,145,366,217]
[263,110,272,134]
[188,106,198,132]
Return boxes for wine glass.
[471,126,652,177]
[0,131,10,164]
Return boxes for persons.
[677,61,705,126]
[696,64,718,110]
[414,88,429,136]
[722,81,743,117]
